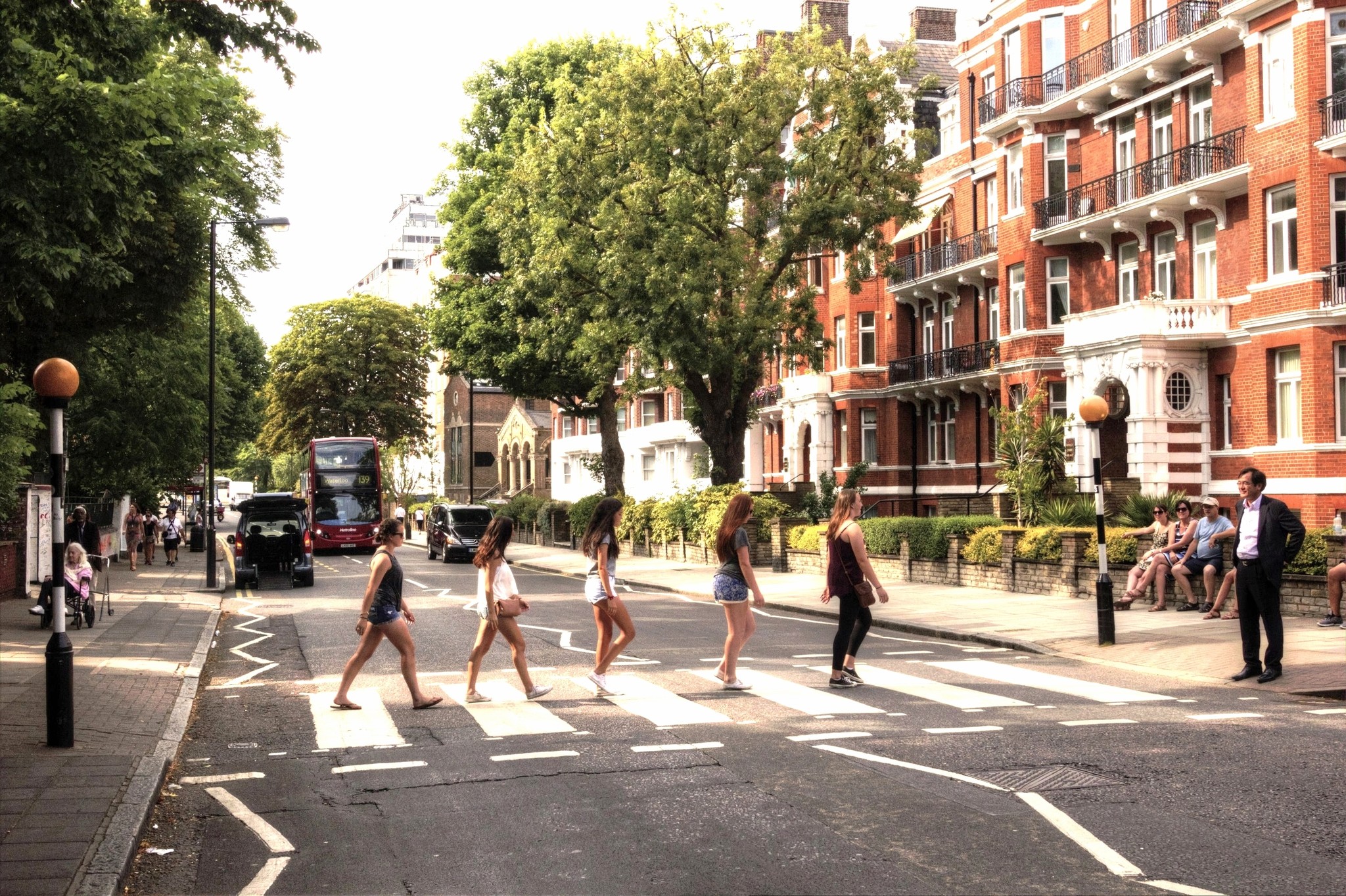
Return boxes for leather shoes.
[1231,666,1283,684]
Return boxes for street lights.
[32,358,80,747]
[206,215,291,588]
[1079,394,1116,646]
[319,408,345,437]
[254,476,258,493]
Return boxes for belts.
[1239,558,1259,566]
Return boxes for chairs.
[280,525,296,553]
[246,525,266,556]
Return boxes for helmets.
[219,502,222,505]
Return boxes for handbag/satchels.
[176,534,181,545]
[162,531,168,538]
[494,598,522,617]
[1142,550,1155,564]
[854,580,876,608]
[136,540,144,553]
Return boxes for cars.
[206,500,219,514]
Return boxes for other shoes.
[71,618,80,626]
[130,555,178,571]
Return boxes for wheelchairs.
[40,575,96,630]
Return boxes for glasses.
[749,509,753,515]
[1152,510,1164,515]
[1202,506,1214,509]
[1237,482,1253,486]
[146,509,150,511]
[146,515,151,517]
[395,532,404,538]
[1176,507,1187,513]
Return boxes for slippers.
[412,696,443,710]
[330,703,362,709]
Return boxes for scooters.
[215,506,226,522]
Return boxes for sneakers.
[65,607,68,614]
[29,605,45,615]
[828,666,864,688]
[525,684,553,700]
[464,691,492,703]
[1316,610,1346,629]
[712,666,753,690]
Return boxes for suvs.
[229,493,253,511]
[226,492,316,590]
[426,502,496,563]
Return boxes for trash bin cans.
[190,526,205,552]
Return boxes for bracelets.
[359,612,368,619]
[607,595,614,600]
[876,585,882,589]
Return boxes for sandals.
[1113,589,1239,620]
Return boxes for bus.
[299,436,388,556]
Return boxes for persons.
[820,488,889,689]
[27,497,223,625]
[1112,504,1174,611]
[1231,467,1306,684]
[1171,497,1236,612]
[463,515,553,703]
[713,494,765,690]
[581,498,636,696]
[1126,500,1199,612]
[1202,567,1239,620]
[329,502,443,710]
[1316,559,1346,629]
